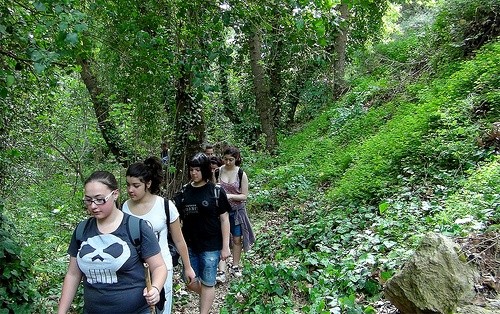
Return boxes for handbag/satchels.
[167,231,181,267]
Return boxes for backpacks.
[75,215,166,311]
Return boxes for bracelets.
[151,286,159,292]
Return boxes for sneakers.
[216,271,226,283]
[231,265,242,278]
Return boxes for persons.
[160,142,222,172]
[176,153,230,314]
[214,148,255,283]
[122,157,195,314]
[56,171,168,314]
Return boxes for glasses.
[82,190,114,205]
